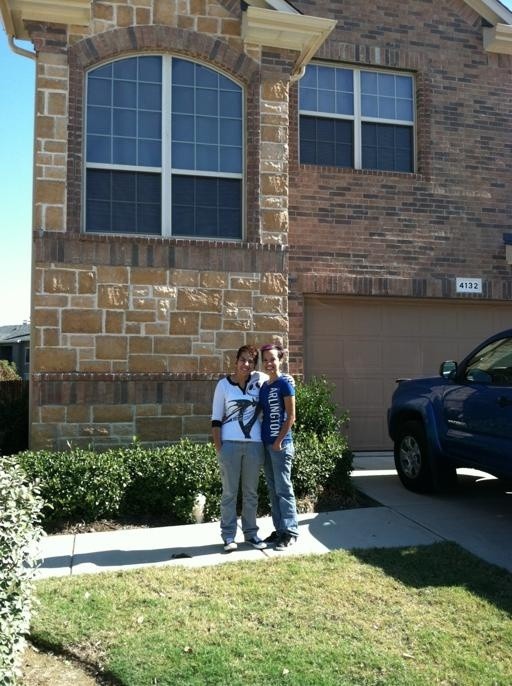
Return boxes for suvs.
[386,329,512,498]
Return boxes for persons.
[259,343,298,551]
[210,345,295,552]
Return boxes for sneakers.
[276,533,297,550]
[264,532,279,545]
[224,541,237,551]
[246,537,266,548]
